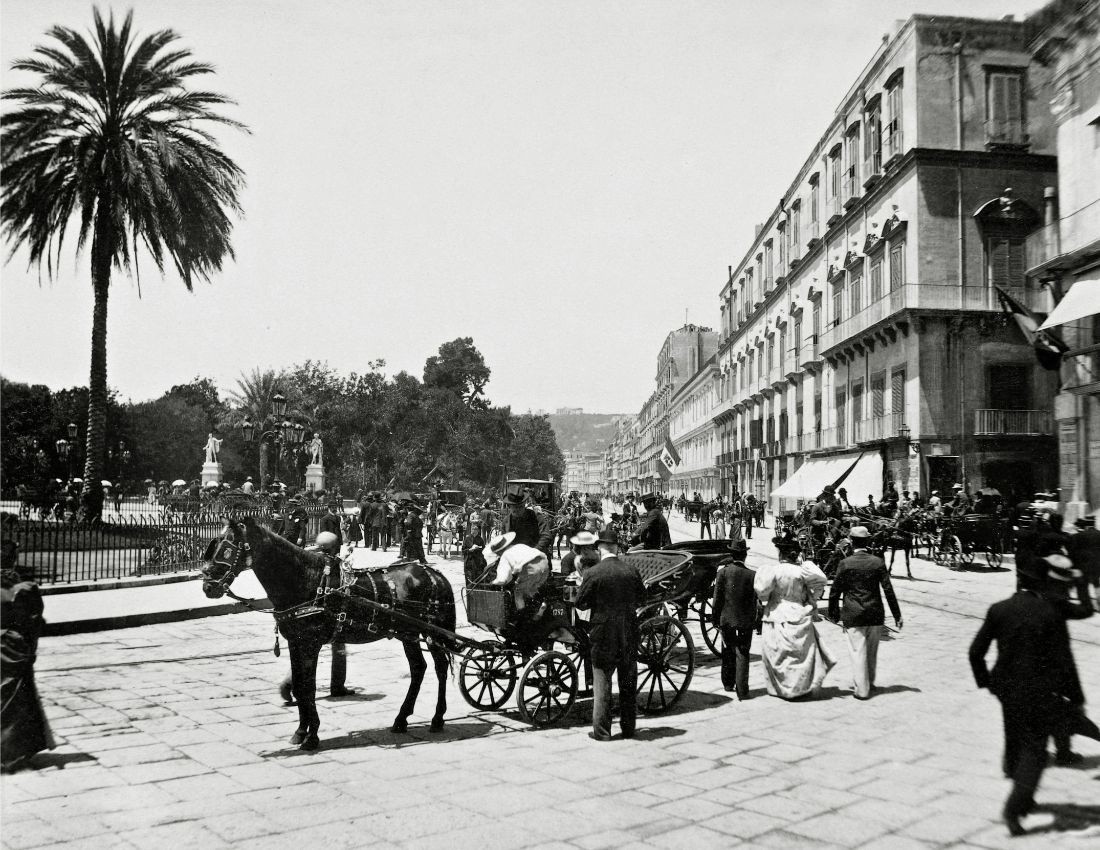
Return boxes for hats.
[610,513,620,518]
[594,530,622,550]
[640,492,655,503]
[1041,555,1077,582]
[952,483,964,489]
[1081,515,1098,521]
[849,526,871,538]
[570,531,599,546]
[1016,558,1051,587]
[726,538,750,552]
[821,485,836,495]
[482,531,516,555]
[502,484,529,505]
[325,500,339,506]
[837,488,847,494]
[626,492,636,499]
[886,480,940,495]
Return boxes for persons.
[277,499,308,550]
[1015,513,1100,612]
[0,540,57,775]
[343,483,672,563]
[203,433,223,464]
[711,526,904,701]
[461,485,552,622]
[999,187,1016,218]
[113,483,122,512]
[560,531,648,742]
[320,500,343,557]
[148,480,230,505]
[804,480,1001,548]
[241,475,254,495]
[673,492,767,539]
[968,554,1100,835]
[310,433,321,464]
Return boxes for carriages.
[771,485,920,586]
[917,486,1010,571]
[198,513,700,757]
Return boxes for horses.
[202,515,457,753]
[396,498,429,567]
[553,506,580,559]
[859,511,920,580]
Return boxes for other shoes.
[331,687,355,697]
[588,732,595,738]
[1003,809,1026,836]
[280,681,292,701]
[1055,751,1084,766]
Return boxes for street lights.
[240,391,307,515]
[106,440,130,512]
[54,422,85,524]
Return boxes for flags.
[657,436,681,482]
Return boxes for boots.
[531,592,547,621]
[516,608,530,628]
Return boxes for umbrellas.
[73,478,83,482]
[144,479,201,487]
[207,481,218,487]
[101,480,112,486]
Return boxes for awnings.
[1036,266,1100,332]
[770,450,863,500]
